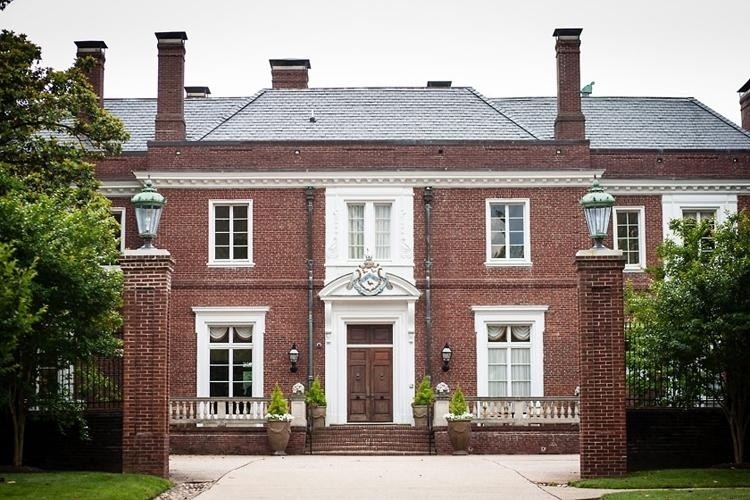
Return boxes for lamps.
[130,175,167,249]
[288,343,299,373]
[441,342,452,372]
[577,175,616,250]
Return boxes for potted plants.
[411,376,437,426]
[446,384,472,455]
[304,375,327,427]
[267,383,292,456]
